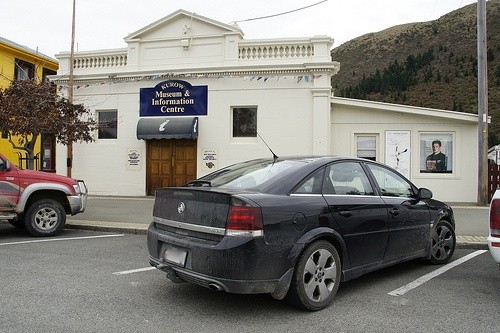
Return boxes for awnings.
[136,116,199,140]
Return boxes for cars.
[147,155,457,311]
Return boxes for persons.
[426,140,448,171]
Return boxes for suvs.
[0,152,90,238]
